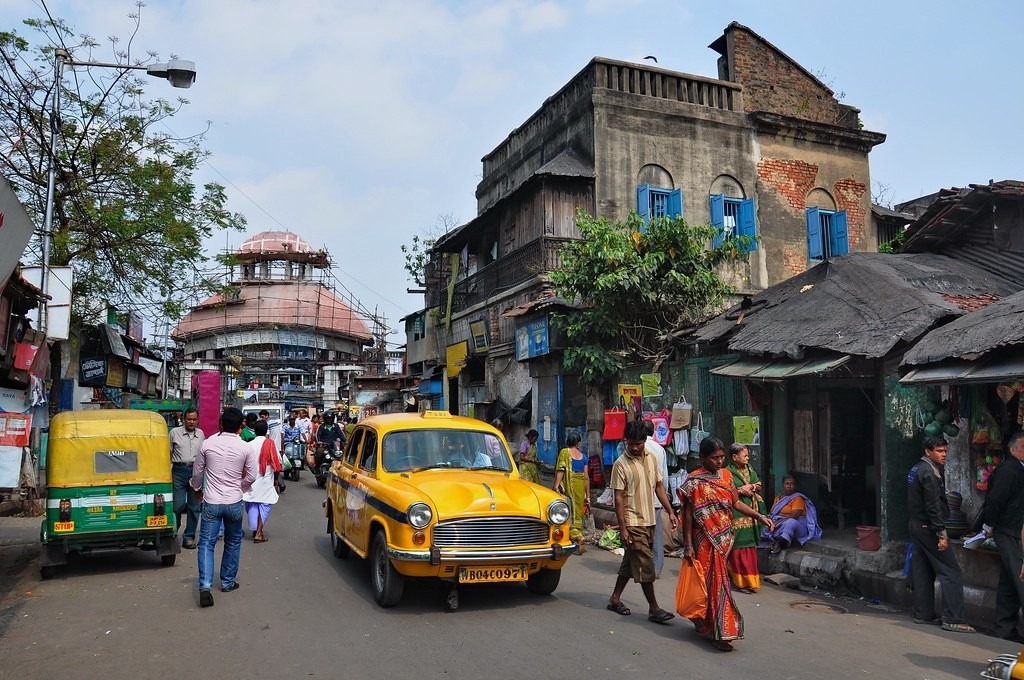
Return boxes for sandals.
[914,618,942,625]
[941,623,976,633]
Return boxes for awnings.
[708,354,878,414]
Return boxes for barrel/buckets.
[856,525,881,551]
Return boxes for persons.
[725,443,761,594]
[484,418,503,467]
[762,474,823,554]
[447,432,493,467]
[168,408,284,608]
[282,410,358,480]
[519,429,544,485]
[552,433,590,556]
[978,430,1024,644]
[607,420,678,618]
[676,437,773,651]
[908,437,976,632]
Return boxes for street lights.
[32,46,199,487]
[160,282,209,400]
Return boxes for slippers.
[607,602,631,615]
[648,611,675,621]
[713,641,732,651]
[254,535,268,543]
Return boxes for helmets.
[289,414,297,422]
[323,411,335,424]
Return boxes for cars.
[321,407,580,609]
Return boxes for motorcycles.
[239,402,345,493]
[37,407,184,579]
[126,398,200,433]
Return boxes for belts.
[174,462,194,467]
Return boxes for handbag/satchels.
[281,453,292,471]
[601,391,710,467]
[583,503,595,535]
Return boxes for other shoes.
[578,537,585,555]
[770,546,782,554]
[182,540,195,548]
[199,589,214,607]
[221,583,239,592]
[279,485,286,492]
